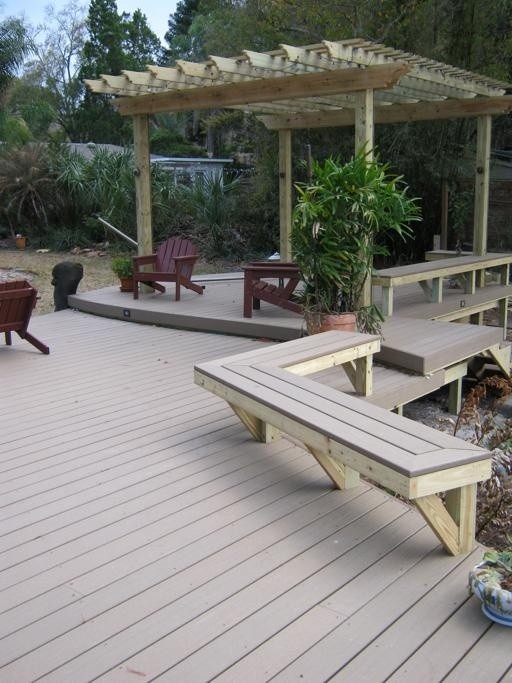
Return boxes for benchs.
[371,248,512,317]
[193,327,496,557]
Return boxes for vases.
[15,237,25,250]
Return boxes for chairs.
[241,261,304,318]
[132,238,203,302]
[0,280,51,355]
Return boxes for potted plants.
[112,257,134,292]
[470,545,512,626]
[289,134,423,341]
